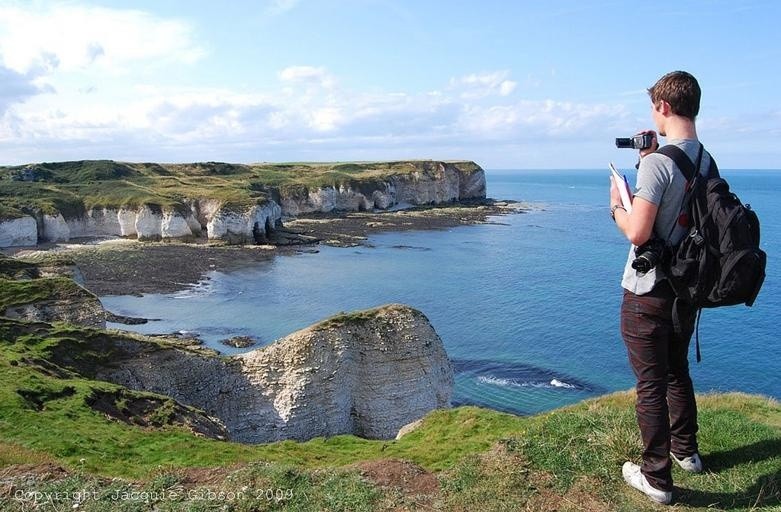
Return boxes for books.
[609,161,634,212]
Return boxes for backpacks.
[652,145,766,308]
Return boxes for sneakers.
[669,438,702,473]
[622,461,673,506]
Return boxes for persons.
[609,65,717,504]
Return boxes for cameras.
[615,133,652,151]
[632,241,669,278]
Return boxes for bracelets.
[610,204,627,222]
